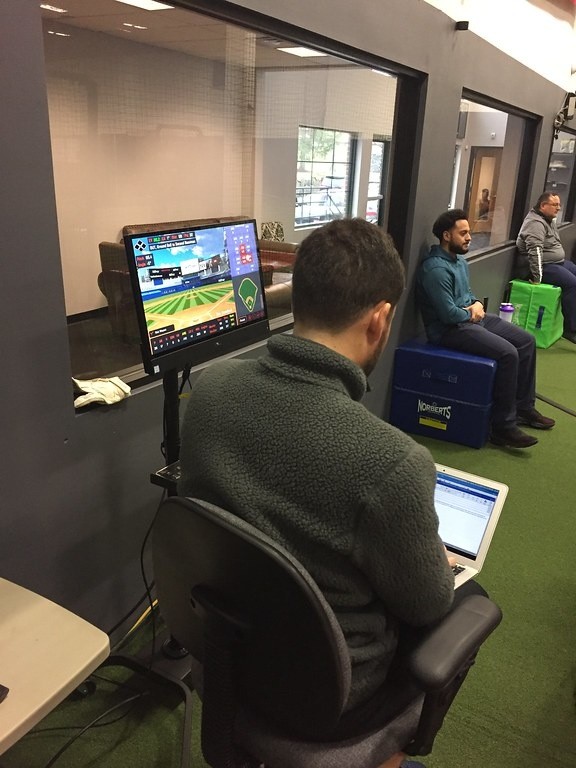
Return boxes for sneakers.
[517,409,555,430]
[490,427,538,448]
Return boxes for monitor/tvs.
[124,220,271,376]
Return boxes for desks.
[0,577,110,756]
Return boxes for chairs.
[149,455,504,767]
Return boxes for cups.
[499,303,514,324]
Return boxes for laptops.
[431,463,508,590]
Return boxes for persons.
[177,216,490,743]
[412,209,555,448]
[516,191,576,345]
